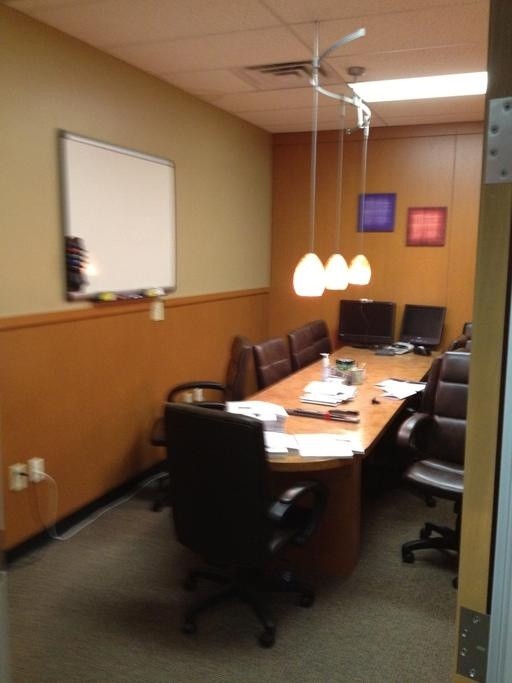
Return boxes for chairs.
[253,334,293,392]
[164,399,322,649]
[285,317,335,374]
[149,331,255,514]
[396,320,473,587]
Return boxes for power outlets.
[28,457,46,483]
[9,464,29,492]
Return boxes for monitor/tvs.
[399,305,448,346]
[338,300,395,347]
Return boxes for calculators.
[384,342,414,355]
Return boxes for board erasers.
[141,288,165,297]
[95,293,118,302]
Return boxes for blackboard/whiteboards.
[59,129,178,303]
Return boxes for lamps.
[291,23,372,296]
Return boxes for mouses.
[413,344,431,356]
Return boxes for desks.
[225,344,443,582]
